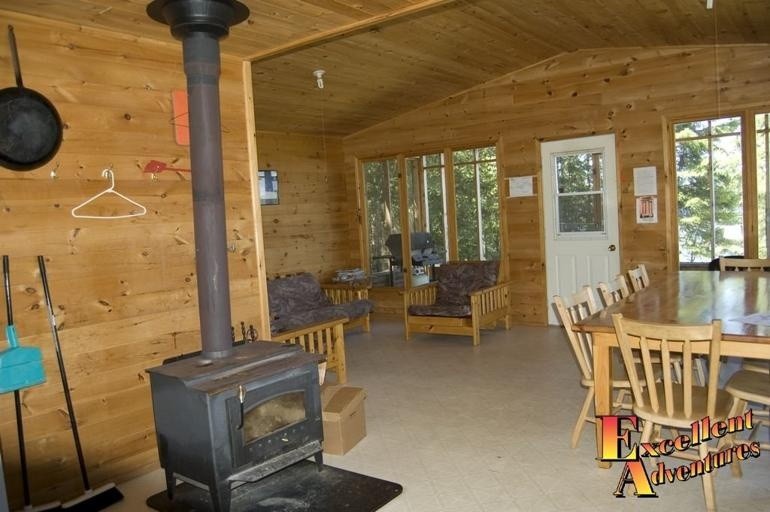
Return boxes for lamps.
[310,68,326,89]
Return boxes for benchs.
[265,272,374,340]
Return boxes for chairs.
[268,310,351,385]
[552,253,770,511]
[404,257,512,345]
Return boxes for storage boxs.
[320,384,367,457]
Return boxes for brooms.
[1,255,125,512]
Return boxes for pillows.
[424,246,433,256]
[411,249,424,262]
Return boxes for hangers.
[70,168,147,220]
[169,105,232,135]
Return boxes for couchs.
[386,230,446,287]
